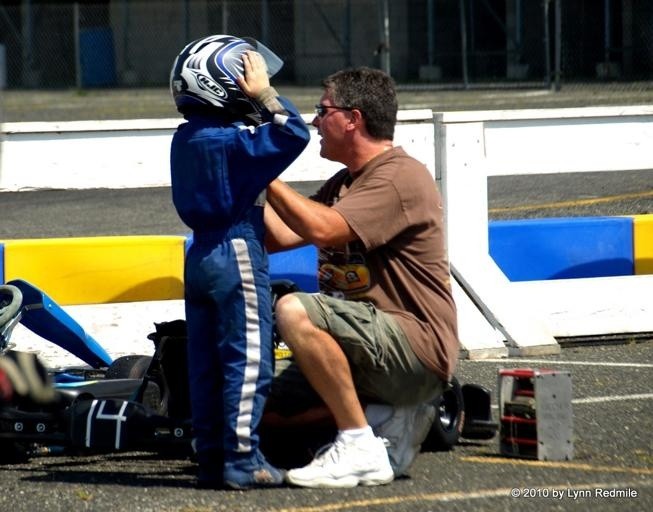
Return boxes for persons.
[165,33,313,492]
[250,65,464,488]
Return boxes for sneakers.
[284,402,436,489]
[197,452,283,490]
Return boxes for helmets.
[169,34,285,118]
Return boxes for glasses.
[315,104,350,117]
